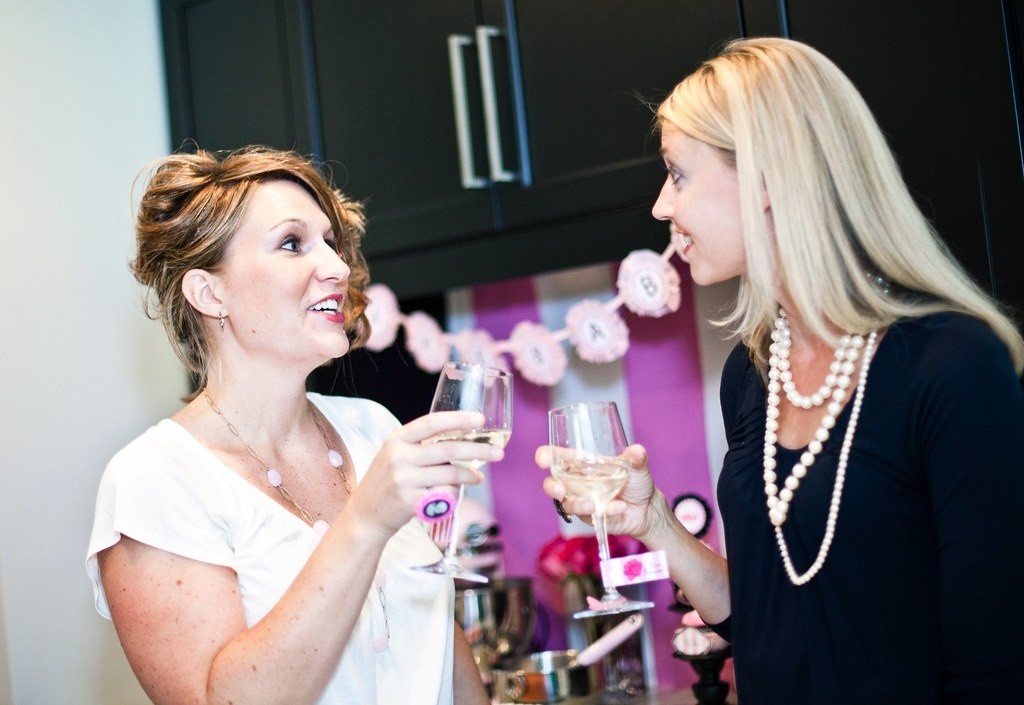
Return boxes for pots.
[490,651,604,705]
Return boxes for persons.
[85,143,506,705]
[534,38,1024,705]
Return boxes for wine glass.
[411,362,514,583]
[548,402,656,619]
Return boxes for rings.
[552,496,572,524]
[418,493,457,523]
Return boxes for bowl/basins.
[453,575,536,681]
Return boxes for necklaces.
[764,308,878,586]
[201,386,390,652]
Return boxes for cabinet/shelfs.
[160,0,1024,312]
